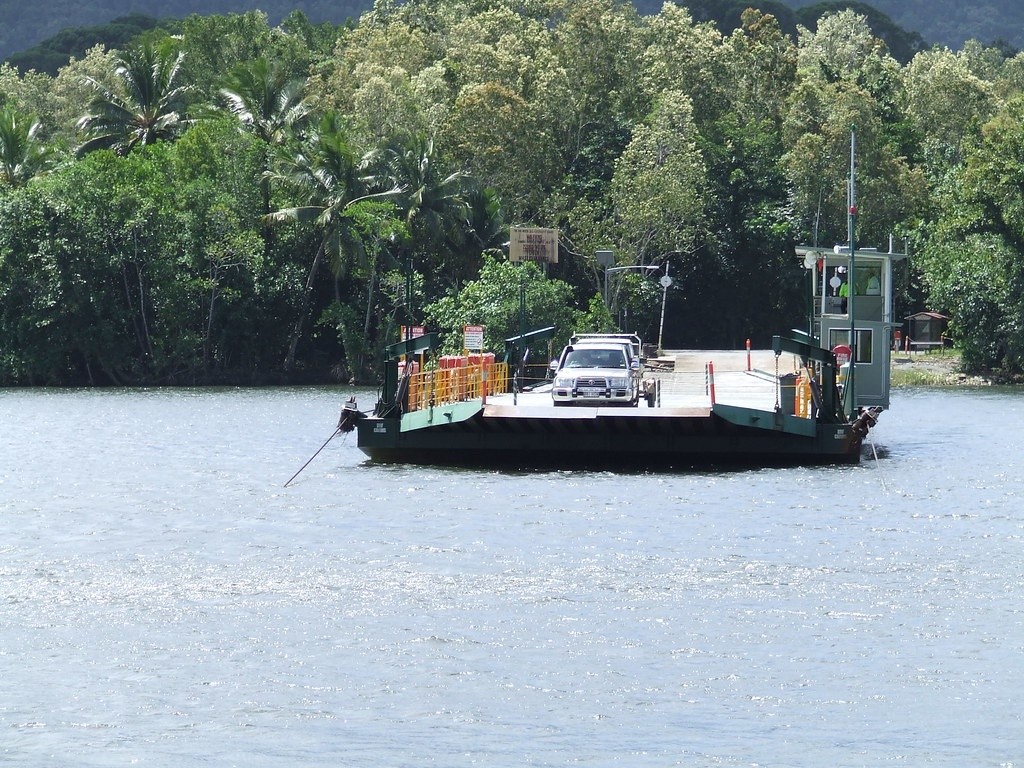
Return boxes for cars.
[577,339,638,362]
[549,343,639,406]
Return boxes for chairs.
[609,352,622,364]
[575,353,592,362]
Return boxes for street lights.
[604,265,659,307]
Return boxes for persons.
[839,277,848,314]
[866,272,879,295]
